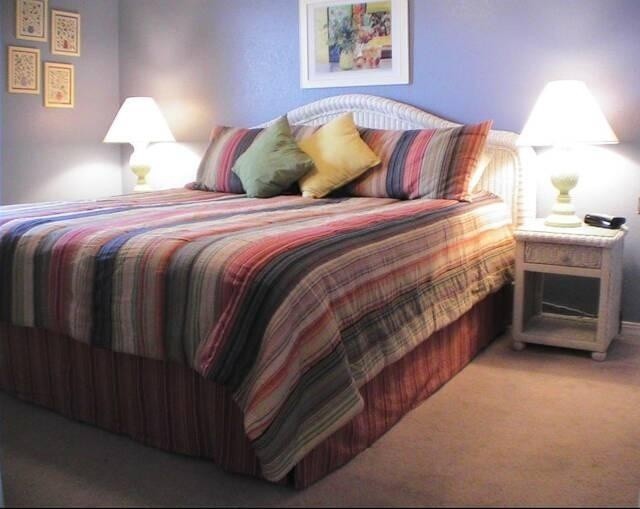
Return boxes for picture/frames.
[8,0,81,108]
[299,0,409,89]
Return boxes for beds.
[0,94,538,490]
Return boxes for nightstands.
[514,218,629,361]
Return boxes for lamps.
[103,97,179,191]
[515,80,619,228]
